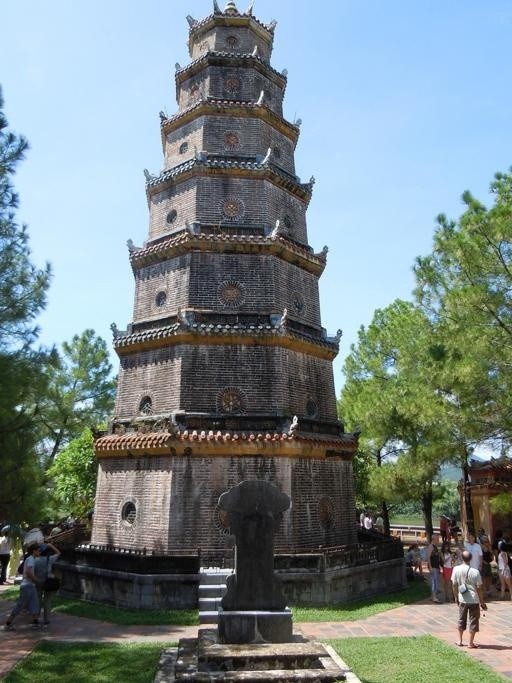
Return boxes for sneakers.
[1,624,15,631]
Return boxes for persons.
[451,550,487,648]
[34,543,61,624]
[5,543,41,631]
[405,515,512,603]
[360,510,385,534]
[0,522,62,585]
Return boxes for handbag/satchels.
[458,584,467,594]
[43,577,60,592]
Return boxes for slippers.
[456,641,479,648]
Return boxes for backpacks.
[406,568,414,581]
